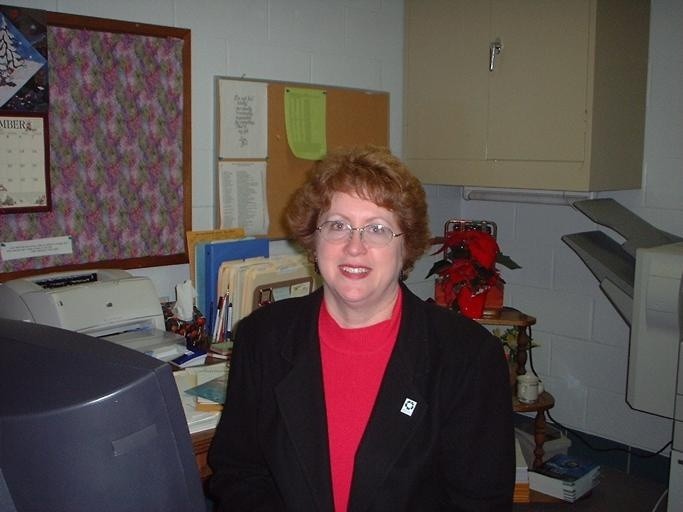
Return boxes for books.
[152,239,319,413]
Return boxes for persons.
[209,143,516,512]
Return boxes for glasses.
[311,219,405,251]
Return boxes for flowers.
[425,229,541,363]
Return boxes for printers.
[0,269,186,364]
[560,198,683,423]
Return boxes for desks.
[166,357,230,481]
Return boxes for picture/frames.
[0,111,52,214]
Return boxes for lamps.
[463,187,597,207]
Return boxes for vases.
[483,276,509,318]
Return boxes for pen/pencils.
[208,290,233,344]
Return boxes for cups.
[517,374,545,404]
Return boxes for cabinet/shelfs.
[452,307,554,471]
[402,0,652,192]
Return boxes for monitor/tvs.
[0,317,208,512]
